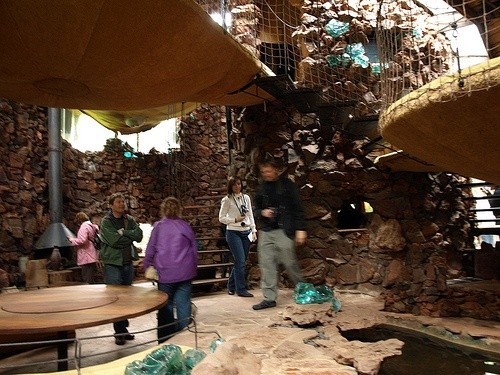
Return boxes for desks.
[0,284,168,372]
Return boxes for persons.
[219,176,257,297]
[66,212,100,285]
[97,193,143,345]
[252,158,308,310]
[143,196,200,344]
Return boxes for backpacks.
[88,223,102,250]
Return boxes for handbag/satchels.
[131,244,139,261]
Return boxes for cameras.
[241,205,249,217]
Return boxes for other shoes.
[125,334,134,340]
[228,290,234,295]
[238,293,253,297]
[115,332,126,345]
[252,300,276,310]
